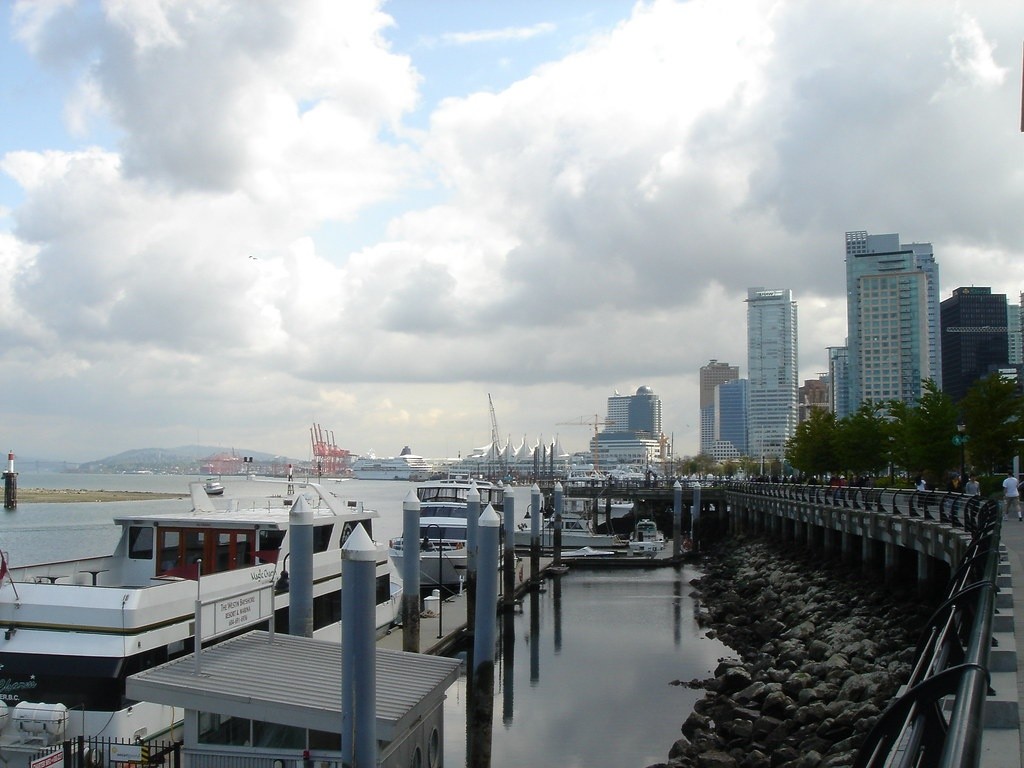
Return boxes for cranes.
[555,413,617,469]
[310,422,349,473]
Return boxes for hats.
[840,475,845,479]
[952,472,960,477]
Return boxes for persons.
[750,474,872,487]
[949,474,962,493]
[915,477,928,490]
[966,473,981,496]
[1002,471,1022,521]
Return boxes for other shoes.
[1019,516,1022,521]
[1003,516,1008,520]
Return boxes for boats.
[388,450,505,600]
[203,472,225,495]
[562,455,605,488]
[352,446,433,481]
[514,484,629,550]
[0,482,404,746]
